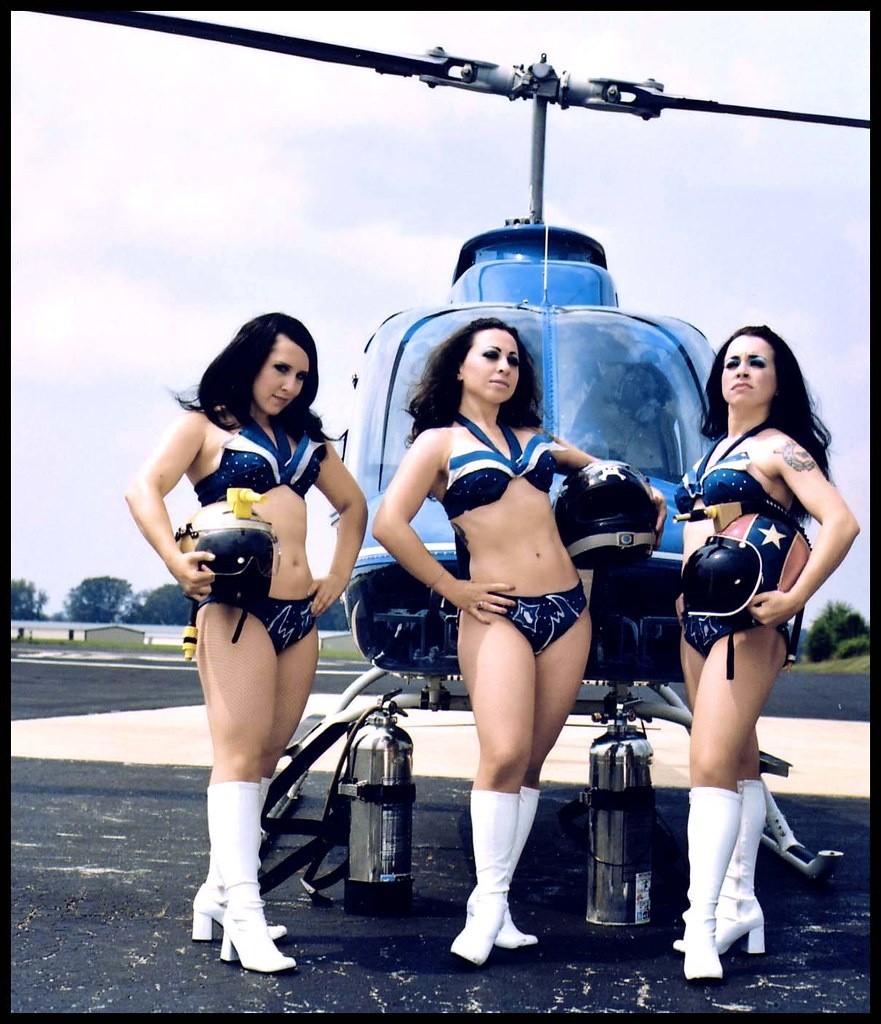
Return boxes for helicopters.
[9,11,869,901]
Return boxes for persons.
[125,312,367,971]
[672,327,860,984]
[372,317,666,966]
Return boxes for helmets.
[548,458,663,560]
[173,488,282,617]
[677,513,813,622]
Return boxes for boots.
[494,785,540,949]
[451,789,523,968]
[682,787,744,984]
[669,779,768,954]
[191,777,289,942]
[204,781,297,974]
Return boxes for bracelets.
[427,569,445,588]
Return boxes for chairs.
[566,363,681,480]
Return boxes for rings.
[478,601,482,609]
